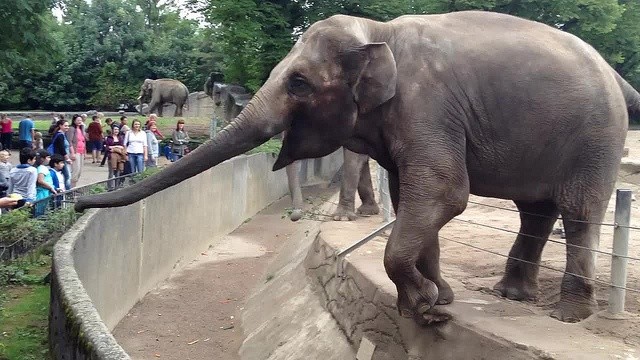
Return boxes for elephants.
[280,125,382,224]
[137,77,191,118]
[72,7,640,325]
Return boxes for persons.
[172,120,190,161]
[0,149,13,196]
[99,130,113,167]
[104,118,121,129]
[59,114,65,120]
[54,120,72,190]
[0,113,13,149]
[120,125,131,174]
[145,114,166,139]
[0,197,29,208]
[84,116,103,163]
[96,113,106,128]
[18,114,35,164]
[66,114,89,188]
[124,119,148,173]
[145,121,158,168]
[120,116,127,124]
[81,114,90,143]
[48,154,66,208]
[35,132,43,151]
[106,124,124,191]
[6,148,38,203]
[36,150,57,216]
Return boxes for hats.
[110,121,121,128]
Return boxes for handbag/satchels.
[123,161,132,174]
[46,143,55,156]
